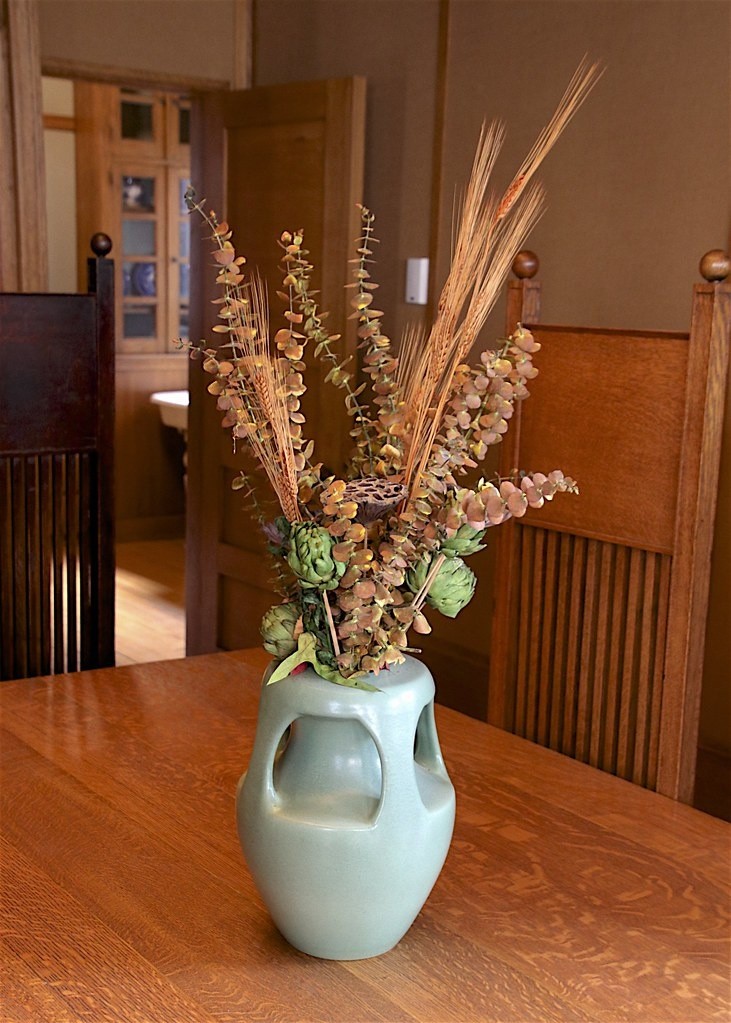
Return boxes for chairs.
[0,231,117,681]
[487,249,731,807]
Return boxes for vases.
[233,653,456,962]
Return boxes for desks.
[0,643,731,1023]
[150,390,189,429]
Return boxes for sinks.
[150,390,189,429]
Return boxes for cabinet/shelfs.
[75,79,193,542]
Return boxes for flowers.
[174,56,613,695]
